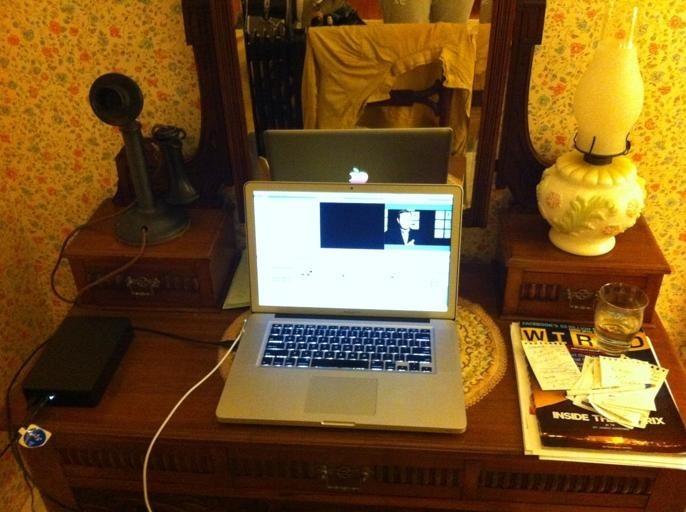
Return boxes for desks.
[0,266,686,512]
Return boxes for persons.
[384,210,422,247]
[311,0,347,27]
[381,1,475,29]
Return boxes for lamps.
[535,7,647,258]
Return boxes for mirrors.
[211,0,516,229]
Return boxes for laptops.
[214,179,468,436]
[263,126,453,185]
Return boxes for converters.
[20,315,135,409]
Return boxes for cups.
[593,281,649,358]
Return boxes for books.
[538,456,686,471]
